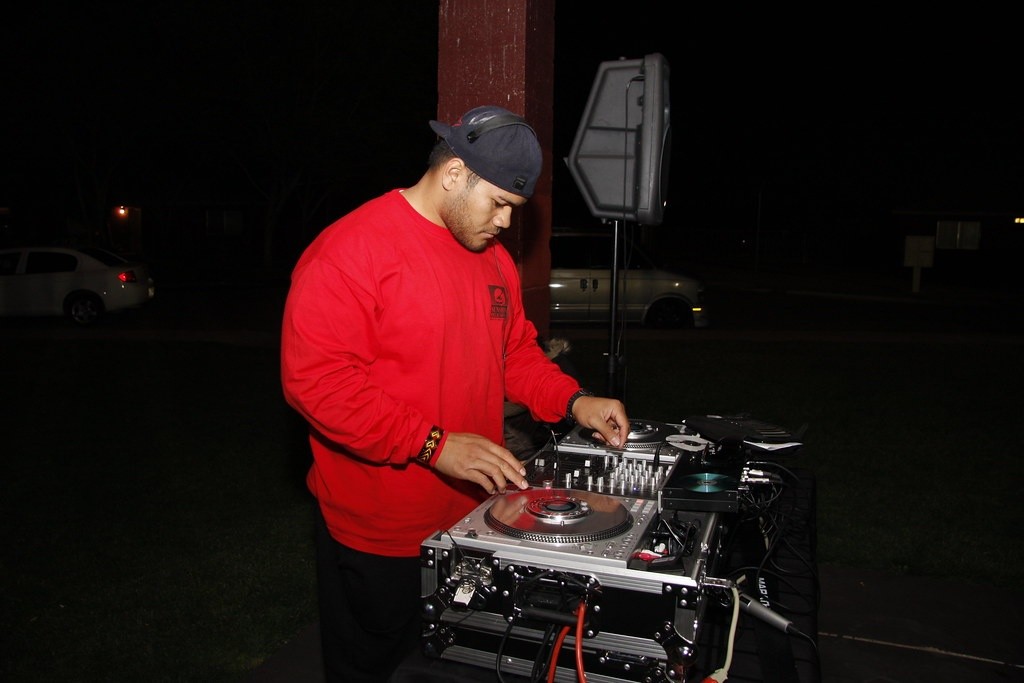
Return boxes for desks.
[390,464,823,683]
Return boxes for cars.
[0,244,155,327]
[548,228,711,332]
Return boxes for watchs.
[567,388,593,424]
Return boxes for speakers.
[569,52,671,227]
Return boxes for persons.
[280,105,629,682]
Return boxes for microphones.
[715,579,801,635]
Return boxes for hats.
[429,106,543,198]
